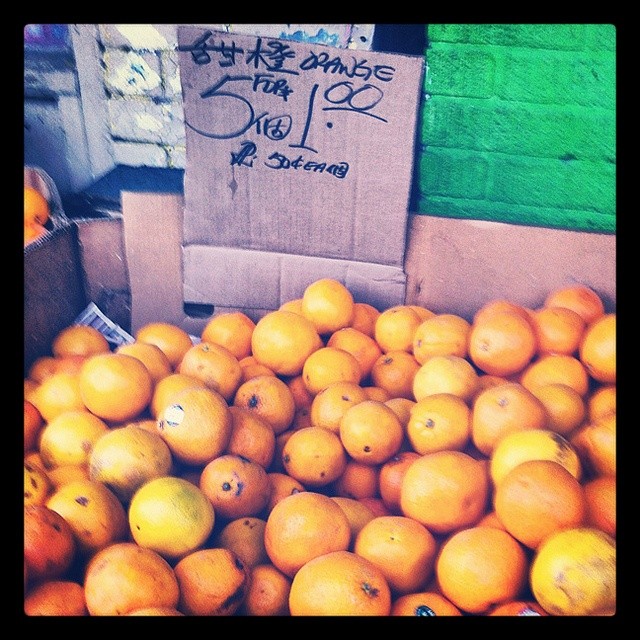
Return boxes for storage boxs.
[23,215,619,617]
